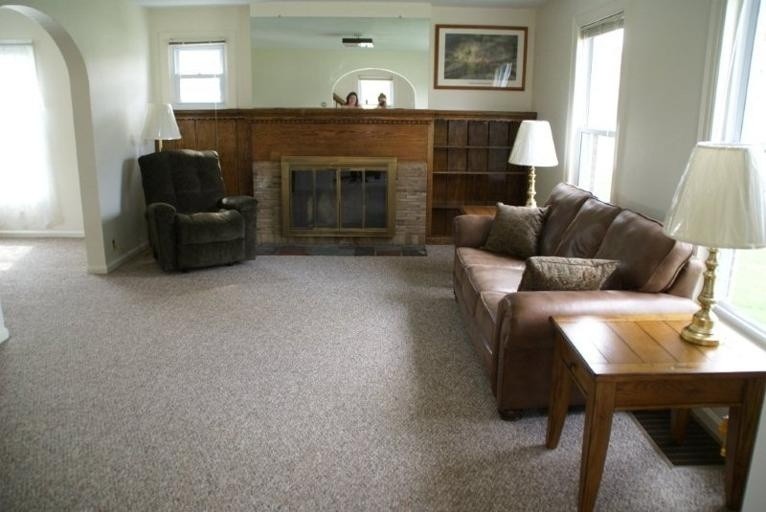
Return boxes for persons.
[378,93,387,106]
[343,92,358,105]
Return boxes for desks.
[543,312,766,510]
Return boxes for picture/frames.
[430,22,530,94]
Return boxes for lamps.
[343,37,373,51]
[661,141,765,349]
[141,102,183,154]
[509,118,562,206]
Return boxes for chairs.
[141,145,249,271]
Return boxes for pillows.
[481,200,620,291]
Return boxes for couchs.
[451,181,707,420]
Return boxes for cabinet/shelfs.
[174,108,535,258]
